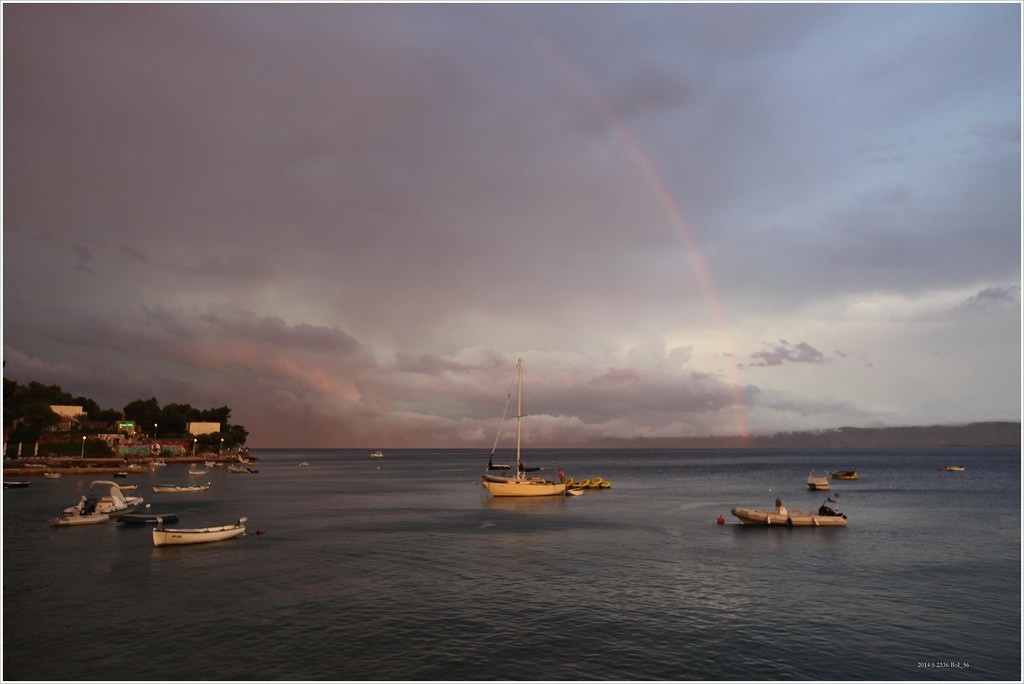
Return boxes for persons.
[557,470,566,483]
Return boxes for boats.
[730,497,846,526]
[299,461,309,466]
[483,481,566,497]
[3,481,31,488]
[50,480,144,526]
[227,465,248,473]
[370,450,384,457]
[189,469,208,474]
[118,510,176,524]
[946,466,964,472]
[114,471,127,478]
[146,516,248,547]
[45,471,60,478]
[124,460,166,472]
[807,468,856,490]
[204,461,222,467]
[151,481,211,493]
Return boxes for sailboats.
[482,359,557,484]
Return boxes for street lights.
[81,436,86,459]
[193,439,198,457]
